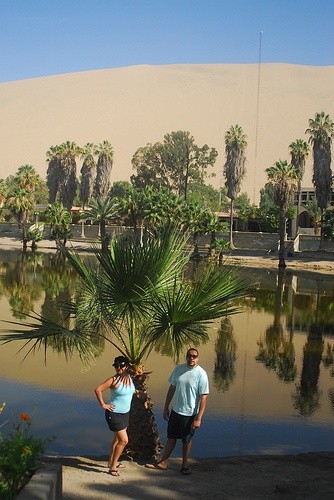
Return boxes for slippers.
[109,469,120,476]
[181,465,192,474]
[146,462,168,470]
[108,462,126,468]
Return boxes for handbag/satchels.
[129,389,153,419]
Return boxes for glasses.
[187,354,198,358]
[114,363,126,368]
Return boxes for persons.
[146,348,209,475]
[95,356,144,476]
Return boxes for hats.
[112,356,129,366]
